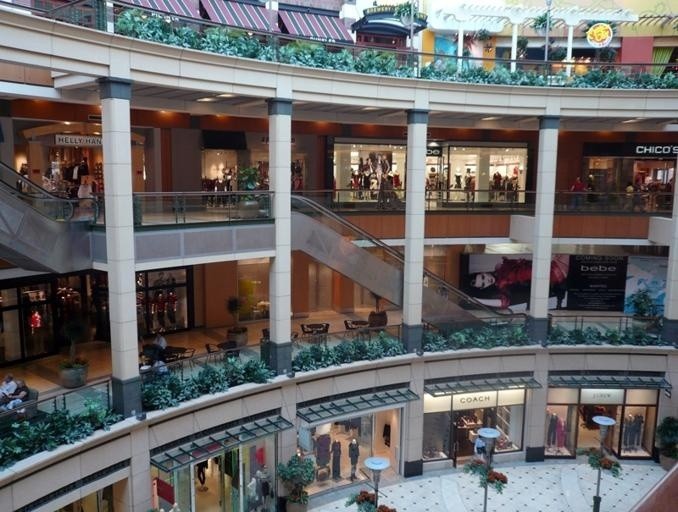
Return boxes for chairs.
[139,318,372,372]
[20,387,39,421]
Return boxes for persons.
[348,438,359,480]
[246,463,273,511]
[625,413,643,450]
[473,436,485,458]
[545,409,566,453]
[60,157,88,185]
[428,168,436,186]
[139,333,166,368]
[492,171,502,201]
[197,461,209,491]
[361,154,519,202]
[30,310,46,354]
[214,180,226,206]
[570,176,673,213]
[329,439,341,479]
[0,373,29,413]
[454,167,471,204]
[151,275,178,332]
[470,253,567,307]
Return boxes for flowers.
[462,456,509,496]
[587,445,624,478]
[286,487,310,508]
[344,490,397,512]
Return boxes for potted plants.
[656,415,678,472]
[276,451,316,512]
[233,167,264,220]
[622,285,665,337]
[222,295,249,345]
[56,312,92,390]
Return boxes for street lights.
[365,456,391,509]
[590,415,615,512]
[474,427,502,512]
[544,0,553,60]
